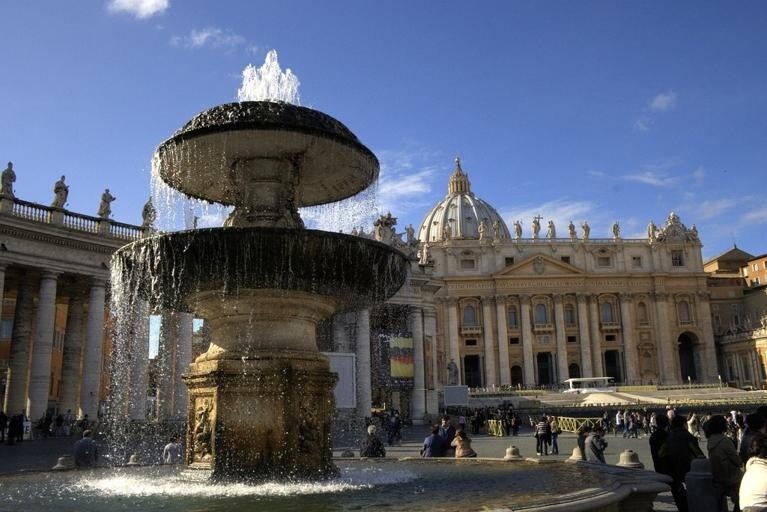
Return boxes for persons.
[0,405,186,468]
[478,219,500,241]
[514,219,556,240]
[404,224,415,241]
[358,402,559,456]
[613,224,620,239]
[1,161,204,239]
[443,223,452,242]
[340,225,405,246]
[577,403,767,512]
[648,211,697,247]
[568,220,590,241]
